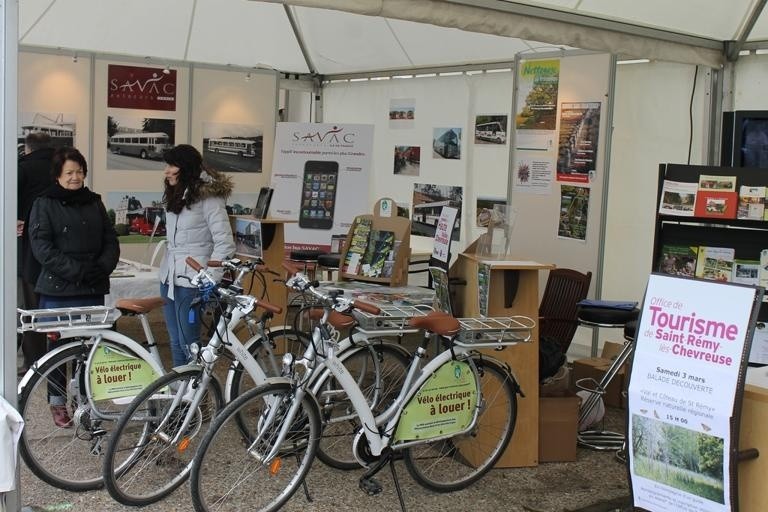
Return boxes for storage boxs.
[573,342,624,409]
[539,383,583,463]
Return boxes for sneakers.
[18,364,33,377]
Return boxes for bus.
[129,207,166,237]
[110,132,170,159]
[413,200,460,229]
[434,130,458,158]
[17,125,74,155]
[209,139,256,157]
[476,122,505,144]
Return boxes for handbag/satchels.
[540,338,563,378]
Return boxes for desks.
[229,215,299,355]
[451,253,556,469]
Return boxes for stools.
[576,307,638,451]
[318,254,340,280]
[615,320,637,464]
[290,250,327,281]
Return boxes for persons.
[17,130,58,378]
[663,252,696,278]
[27,146,121,429]
[149,143,237,437]
[570,191,588,227]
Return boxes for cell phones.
[299,160,339,230]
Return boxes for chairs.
[539,268,592,382]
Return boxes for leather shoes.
[72,399,91,428]
[50,402,72,428]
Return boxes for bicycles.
[16,251,338,492]
[190,261,536,512]
[100,256,422,508]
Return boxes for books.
[341,217,403,278]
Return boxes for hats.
[164,145,202,169]
